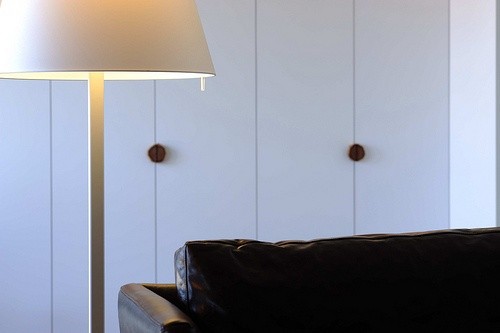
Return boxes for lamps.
[0,0,217,332]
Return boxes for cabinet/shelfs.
[1,0,449,331]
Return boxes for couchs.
[118,224,500,332]
